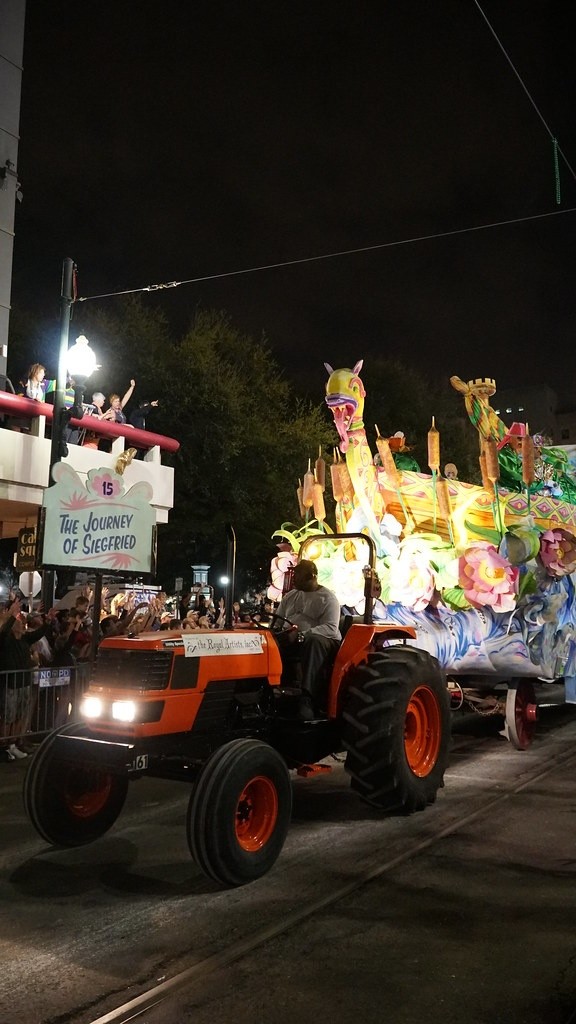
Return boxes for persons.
[0,587,277,761]
[497,423,576,498]
[271,560,342,721]
[374,431,420,472]
[17,364,159,460]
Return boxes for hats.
[161,612,174,620]
[287,560,317,574]
[27,611,44,618]
[187,610,200,617]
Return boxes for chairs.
[287,614,353,668]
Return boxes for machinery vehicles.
[24,525,567,891]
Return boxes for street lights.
[40,334,97,613]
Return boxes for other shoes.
[9,744,28,759]
[2,746,15,762]
[297,698,313,720]
[21,744,33,754]
